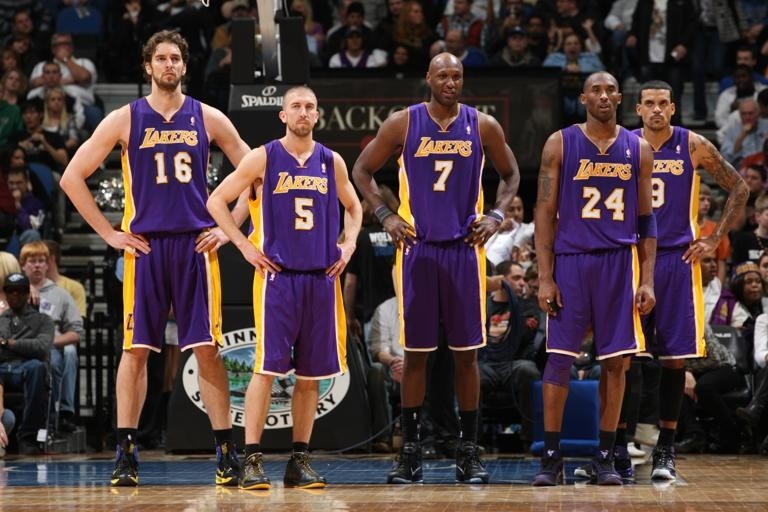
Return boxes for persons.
[1,0,767,487]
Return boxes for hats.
[3,273,29,288]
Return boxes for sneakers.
[455,440,489,483]
[590,450,623,485]
[533,447,563,485]
[214,442,242,485]
[110,440,139,486]
[388,444,424,484]
[615,445,636,484]
[649,445,676,479]
[284,448,326,489]
[238,450,271,490]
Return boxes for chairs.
[351,292,764,455]
